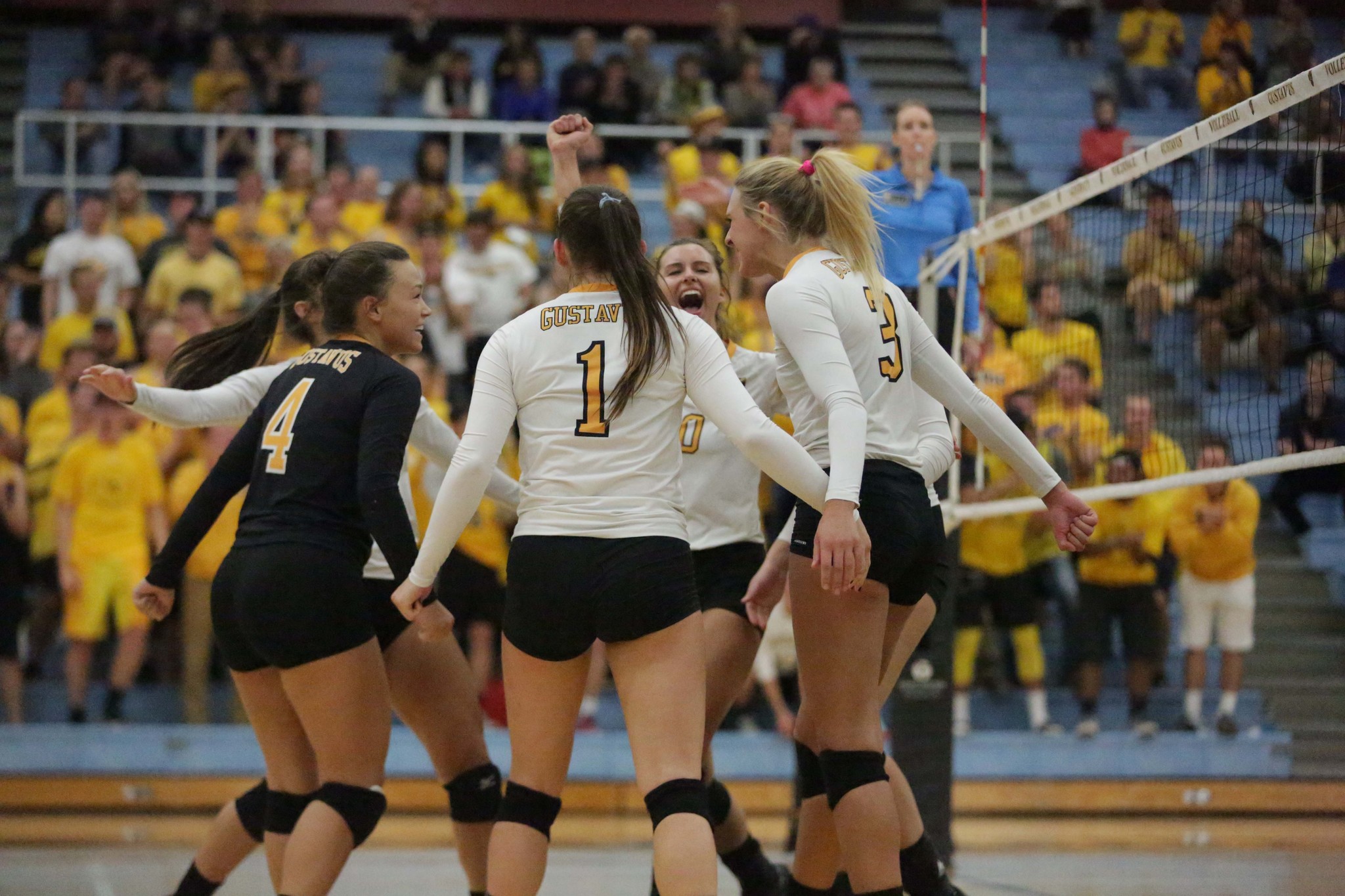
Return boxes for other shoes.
[1077,718,1098,738]
[1217,712,1238,732]
[1131,716,1160,735]
[1176,714,1196,731]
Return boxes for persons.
[389,182,871,896]
[851,99,981,427]
[0,0,1345,896]
